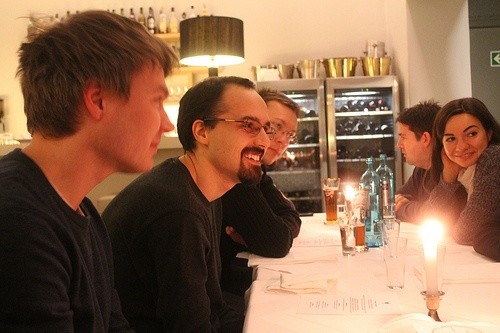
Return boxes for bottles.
[28,4,208,37]
[264,99,393,213]
[376,154,395,219]
[359,158,384,248]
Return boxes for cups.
[277,40,392,79]
[321,178,409,290]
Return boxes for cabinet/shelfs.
[256,75,403,216]
[155,33,207,75]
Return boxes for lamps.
[179,15,245,77]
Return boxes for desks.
[242,213,500,333]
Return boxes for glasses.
[272,121,297,141]
[200,118,276,140]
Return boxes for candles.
[422,232,438,293]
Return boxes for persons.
[216,87,302,294]
[102,76,275,333]
[419,98,500,262]
[0,10,179,333]
[394,100,443,224]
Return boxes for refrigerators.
[254,73,404,214]
